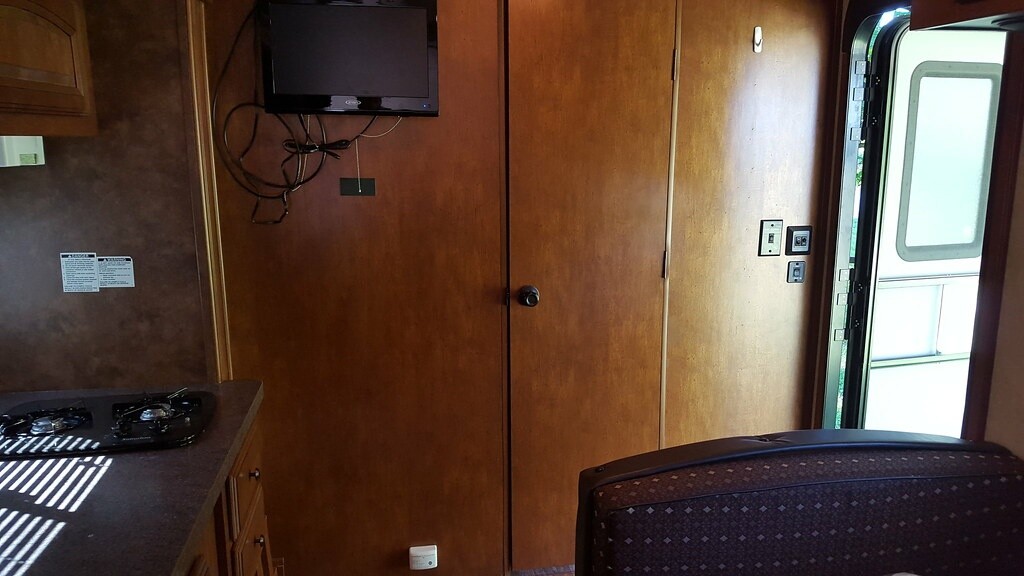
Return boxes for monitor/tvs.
[260,0,439,116]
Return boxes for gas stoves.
[0,391,219,459]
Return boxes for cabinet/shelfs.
[184,407,282,576]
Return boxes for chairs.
[498,427,1024,576]
